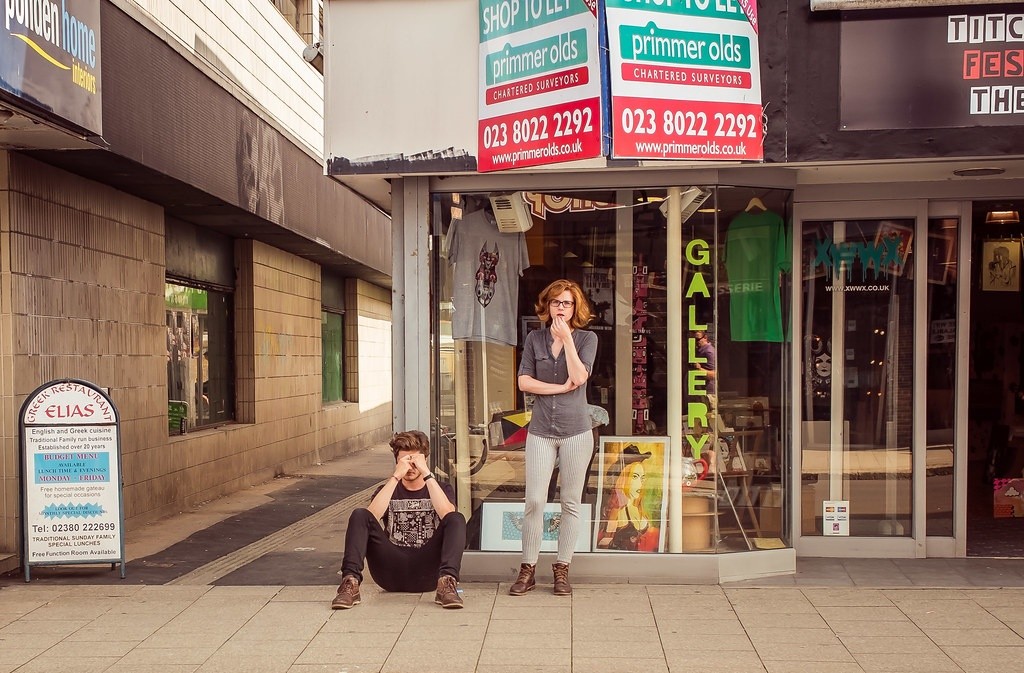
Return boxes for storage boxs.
[993,476,1024,518]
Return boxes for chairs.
[681,429,763,538]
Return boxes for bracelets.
[391,474,401,483]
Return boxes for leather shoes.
[433,576,464,608]
[552,563,572,594]
[509,563,536,595]
[331,575,361,609]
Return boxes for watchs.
[424,472,436,481]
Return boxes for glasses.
[548,298,574,309]
[690,335,705,342]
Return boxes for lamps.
[658,185,711,226]
[303,41,323,63]
[985,200,1020,225]
[489,191,534,234]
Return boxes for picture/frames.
[868,220,914,277]
[479,500,593,553]
[681,412,748,474]
[907,233,954,286]
[591,435,671,552]
[980,237,1022,293]
[802,227,827,282]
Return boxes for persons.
[332,430,466,609]
[694,328,717,418]
[203,349,209,396]
[511,280,594,596]
